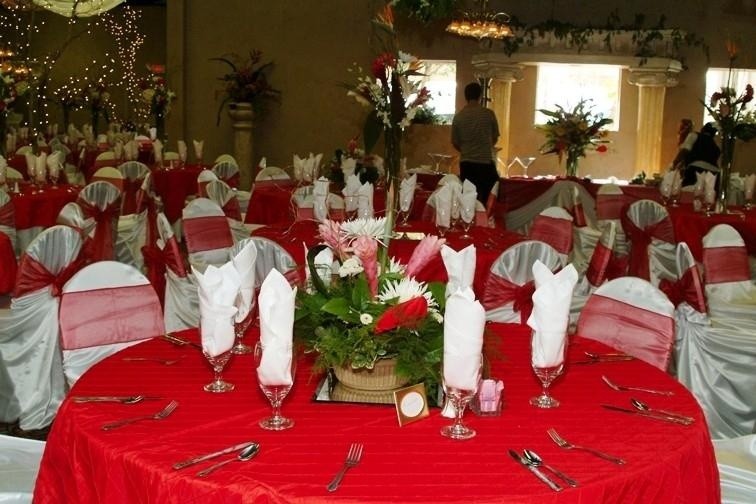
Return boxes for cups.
[294,168,314,186]
[474,378,502,416]
[311,193,372,221]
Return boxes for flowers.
[0,50,176,120]
[337,50,446,175]
[534,99,614,177]
[699,82,755,204]
[205,47,283,127]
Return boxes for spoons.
[584,351,627,360]
[630,398,696,422]
[198,443,259,476]
[522,448,577,487]
[71,394,142,403]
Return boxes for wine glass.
[530,331,569,408]
[435,204,476,241]
[27,167,59,194]
[399,196,414,226]
[661,184,716,217]
[745,190,753,210]
[440,354,483,439]
[232,288,257,354]
[252,340,298,430]
[155,149,203,172]
[426,152,458,172]
[198,314,236,392]
[495,155,537,178]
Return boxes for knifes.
[173,441,254,470]
[572,356,632,364]
[507,449,563,492]
[163,332,201,348]
[601,405,689,424]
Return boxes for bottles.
[441,394,457,418]
[12,181,20,193]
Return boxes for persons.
[453,83,501,209]
[678,117,694,144]
[669,122,721,201]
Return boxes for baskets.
[329,347,414,393]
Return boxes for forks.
[546,428,626,465]
[102,398,179,431]
[122,354,184,365]
[326,441,364,492]
[602,375,674,397]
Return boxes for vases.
[228,102,258,130]
